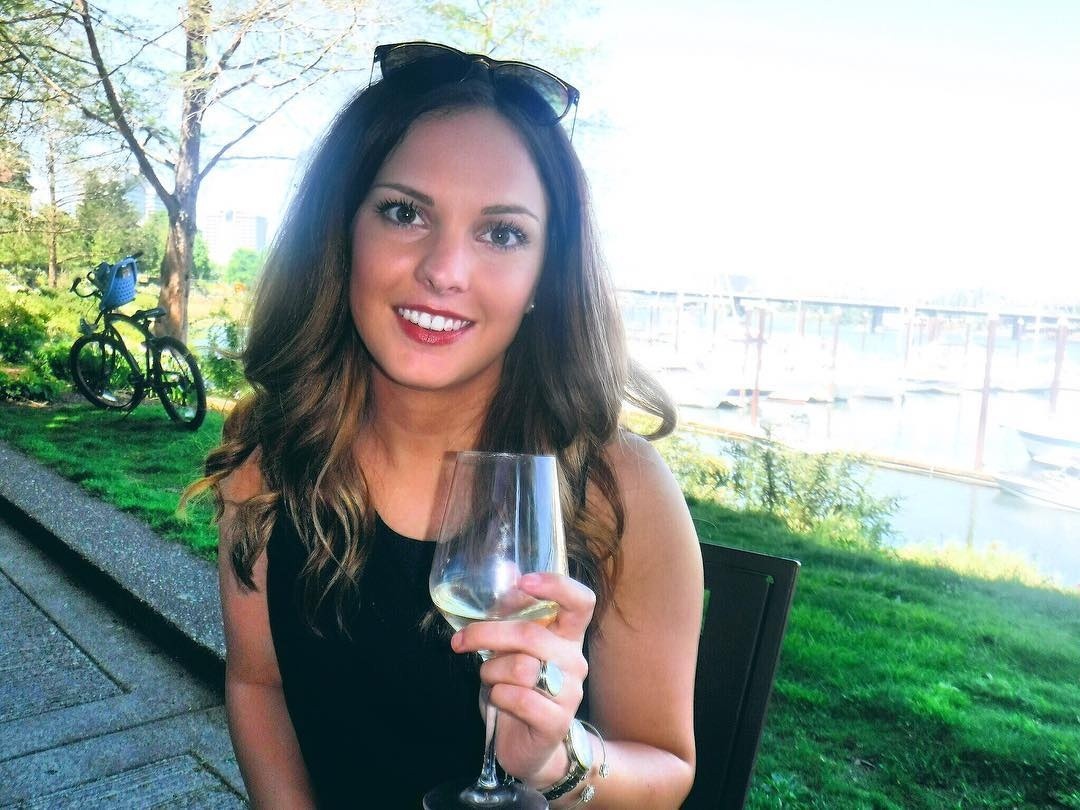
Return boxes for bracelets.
[568,721,609,810]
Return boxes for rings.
[534,661,565,697]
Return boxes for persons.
[173,40,703,810]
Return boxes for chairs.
[567,529,800,810]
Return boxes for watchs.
[538,718,592,800]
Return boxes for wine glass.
[421,452,572,810]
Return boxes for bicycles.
[68,250,207,432]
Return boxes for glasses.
[368,41,581,146]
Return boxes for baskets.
[88,261,137,307]
